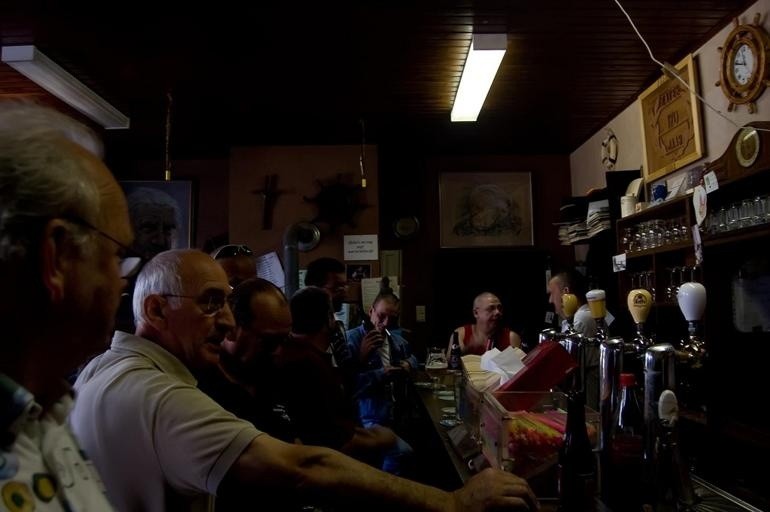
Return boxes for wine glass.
[425,347,447,392]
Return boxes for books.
[557,205,611,246]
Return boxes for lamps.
[449,32,506,123]
[0,44,130,130]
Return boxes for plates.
[734,124,759,168]
[437,389,455,402]
[415,382,447,390]
[557,207,610,247]
[438,406,457,427]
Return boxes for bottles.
[554,388,598,512]
[619,214,688,255]
[623,265,694,305]
[448,329,464,371]
[604,371,649,512]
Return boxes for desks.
[410,371,766,512]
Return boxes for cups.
[697,193,770,234]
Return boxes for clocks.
[713,13,770,114]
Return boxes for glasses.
[215,244,254,261]
[162,287,237,314]
[68,215,143,279]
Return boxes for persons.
[1,109,543,512]
[547,270,612,411]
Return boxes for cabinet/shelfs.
[612,121,770,512]
[546,170,645,331]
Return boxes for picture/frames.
[637,53,706,184]
[439,172,534,249]
